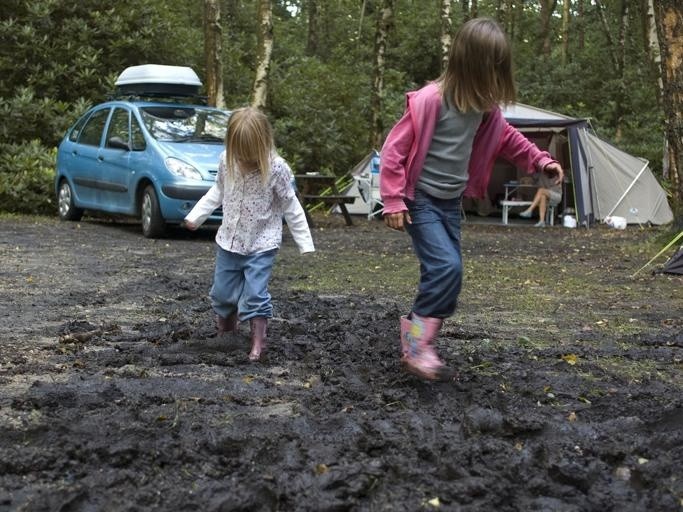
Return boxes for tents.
[326,97,675,227]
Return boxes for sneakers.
[520,211,532,217]
[534,222,546,227]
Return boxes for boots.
[400,311,447,382]
[248,316,267,359]
[216,312,237,332]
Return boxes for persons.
[183,106,316,361]
[360,17,564,381]
[519,156,563,228]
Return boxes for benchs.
[498,199,558,226]
[300,195,360,228]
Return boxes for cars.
[51,99,300,239]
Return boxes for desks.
[502,182,573,208]
[294,174,354,227]
